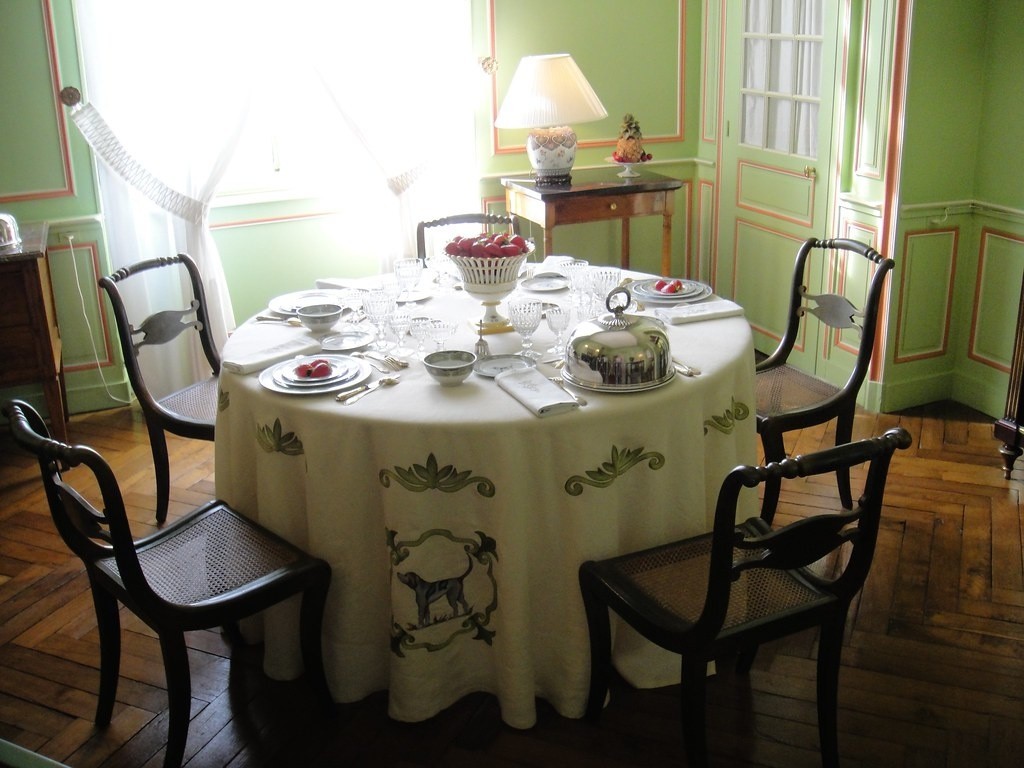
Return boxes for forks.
[363,350,404,372]
[548,377,588,407]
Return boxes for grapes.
[612,152,652,163]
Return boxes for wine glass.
[394,259,423,307]
[559,260,589,314]
[589,267,622,322]
[510,299,542,360]
[340,290,455,363]
[545,307,568,355]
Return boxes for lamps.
[494,53,609,184]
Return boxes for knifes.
[336,373,400,401]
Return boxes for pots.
[559,287,677,393]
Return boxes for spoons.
[343,378,398,406]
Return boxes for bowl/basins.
[297,303,342,334]
[423,349,478,387]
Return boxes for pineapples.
[615,112,644,162]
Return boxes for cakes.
[566,319,671,385]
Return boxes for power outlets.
[58,230,80,244]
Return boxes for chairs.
[416,213,519,261]
[97,253,222,525]
[579,426,912,768]
[5,398,334,768]
[757,235,895,522]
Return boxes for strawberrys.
[655,280,682,293]
[443,230,529,274]
[293,359,331,379]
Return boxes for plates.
[621,276,713,306]
[520,277,570,291]
[259,353,372,395]
[268,288,354,318]
[472,353,538,378]
[320,328,373,352]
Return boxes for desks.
[209,264,758,731]
[0,220,69,450]
[501,166,684,277]
